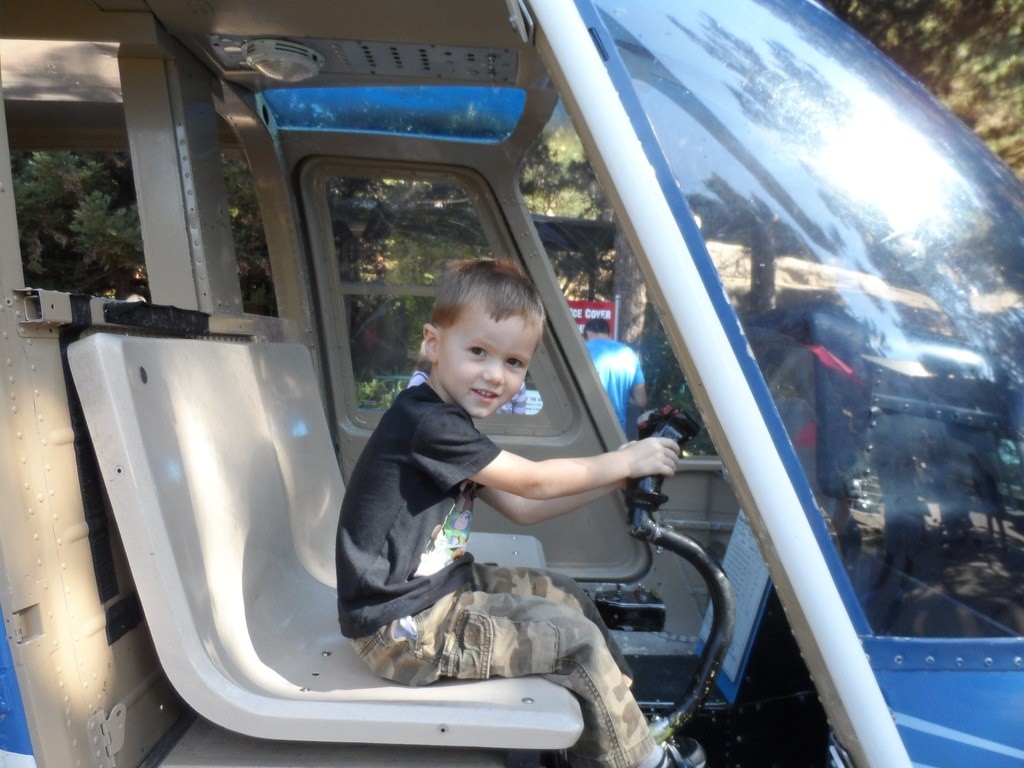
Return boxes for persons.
[584,318,647,430]
[336,258,705,768]
[407,340,527,414]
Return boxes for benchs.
[66,331,587,751]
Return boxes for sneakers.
[654,737,706,768]
